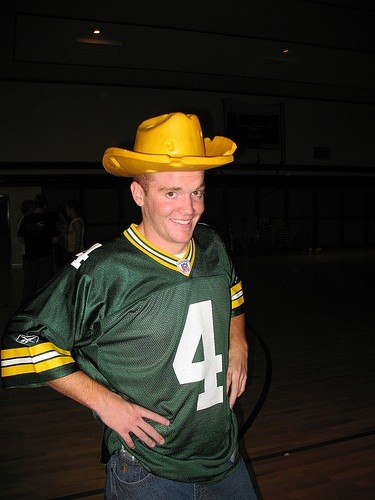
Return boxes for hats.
[103,111,237,177]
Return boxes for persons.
[0,112,259,500]
[16,194,106,305]
